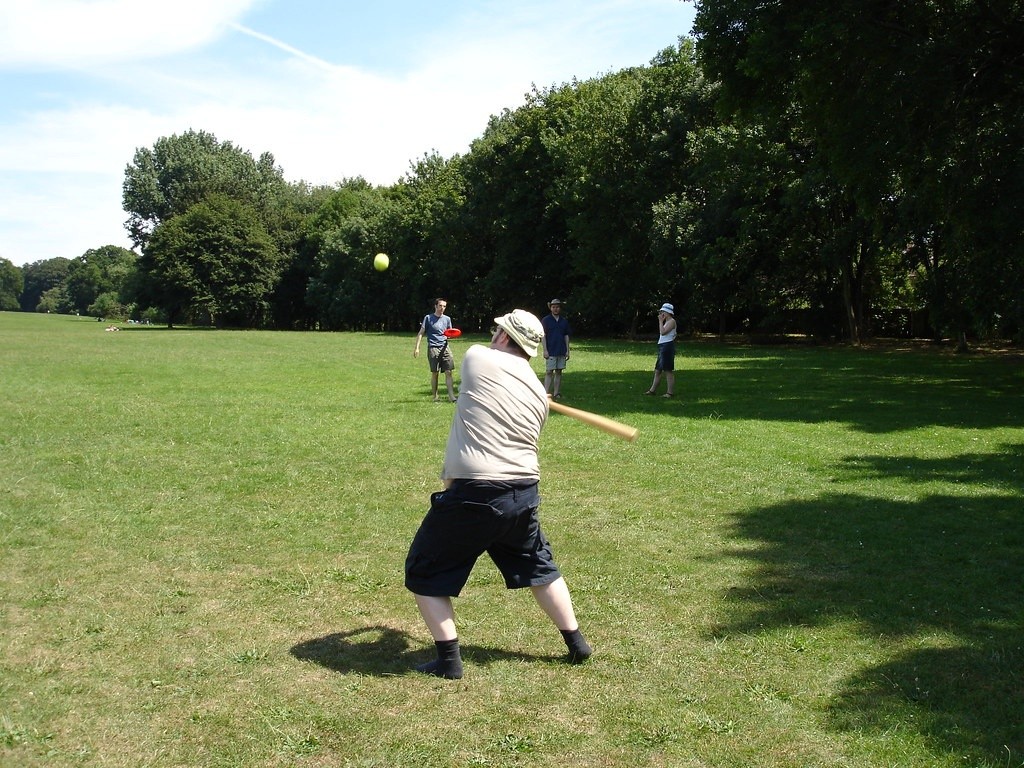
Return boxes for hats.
[660,303,674,315]
[547,299,562,308]
[493,309,544,358]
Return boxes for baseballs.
[373,252,389,271]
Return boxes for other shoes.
[554,393,562,401]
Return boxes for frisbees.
[445,329,461,336]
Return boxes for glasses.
[490,327,502,334]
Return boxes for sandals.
[644,390,655,395]
[663,393,673,398]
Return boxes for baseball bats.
[543,398,638,440]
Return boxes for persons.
[123,319,153,326]
[643,303,677,397]
[97,318,106,322]
[414,298,457,402]
[541,299,569,398]
[106,325,121,331]
[403,309,591,679]
